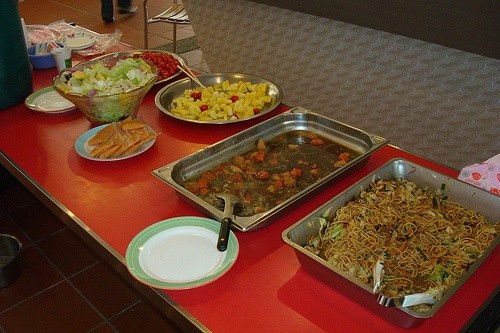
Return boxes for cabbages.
[56,56,157,122]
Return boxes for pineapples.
[170,80,272,121]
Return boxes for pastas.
[310,183,500,294]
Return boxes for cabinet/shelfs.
[144,0,191,52]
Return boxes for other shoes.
[119,7,135,14]
[104,16,117,25]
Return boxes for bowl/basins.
[155,72,284,125]
[0,234,22,288]
[27,42,64,69]
[52,58,159,127]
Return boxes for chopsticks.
[176,62,206,89]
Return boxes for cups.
[51,47,73,73]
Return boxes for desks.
[0,22,500,333]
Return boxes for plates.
[74,123,156,161]
[25,86,75,113]
[62,33,95,51]
[125,216,239,290]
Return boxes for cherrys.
[122,51,181,80]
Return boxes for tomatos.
[189,135,350,212]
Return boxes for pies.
[88,116,155,159]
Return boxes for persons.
[100,0,139,25]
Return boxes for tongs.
[371,252,442,308]
